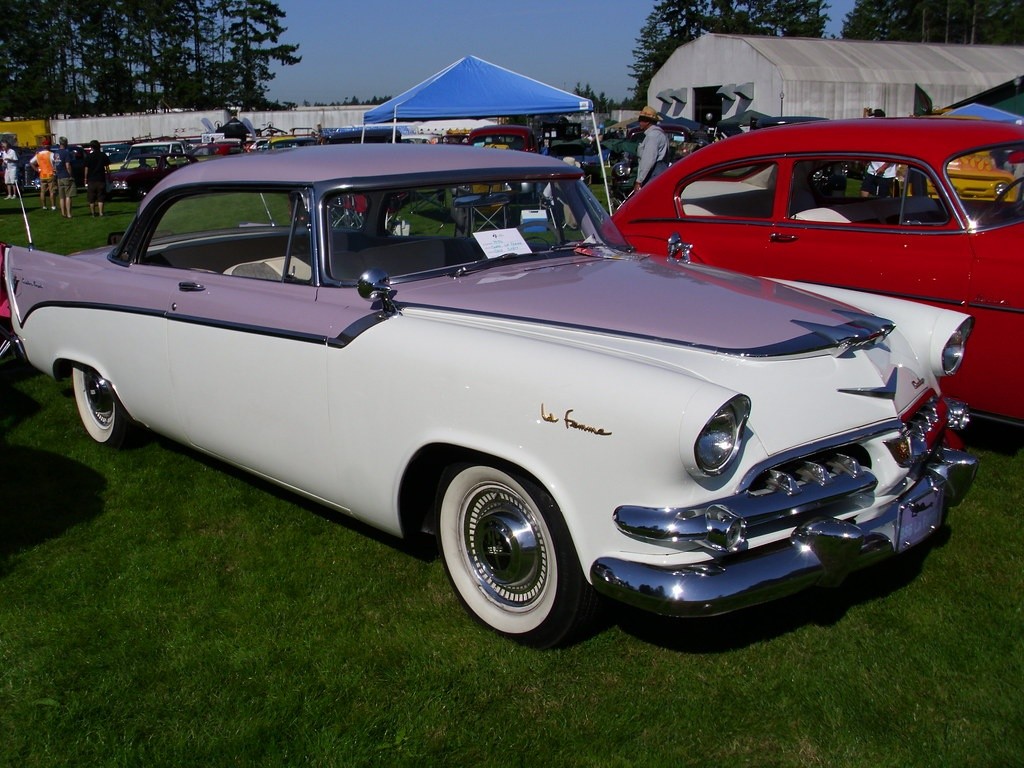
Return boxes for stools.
[453,193,508,239]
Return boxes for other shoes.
[43,207,48,210]
[99,214,103,216]
[11,196,16,199]
[91,215,95,217]
[67,215,72,218]
[61,215,67,218]
[4,196,12,200]
[51,206,56,210]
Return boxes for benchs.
[681,189,812,220]
[791,196,946,225]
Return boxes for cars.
[611,123,710,200]
[579,117,1023,430]
[464,126,550,158]
[890,148,1021,207]
[3,145,979,652]
[11,125,469,203]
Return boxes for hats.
[40,140,50,146]
[634,106,658,120]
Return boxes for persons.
[309,131,326,145]
[910,167,928,196]
[860,108,896,196]
[0,242,14,359]
[207,138,217,154]
[29,137,111,218]
[581,130,592,140]
[544,157,581,214]
[632,106,667,193]
[0,140,19,200]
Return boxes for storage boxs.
[521,209,548,232]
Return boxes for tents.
[360,55,612,216]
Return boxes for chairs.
[0,241,26,362]
[224,255,313,283]
[538,192,568,228]
